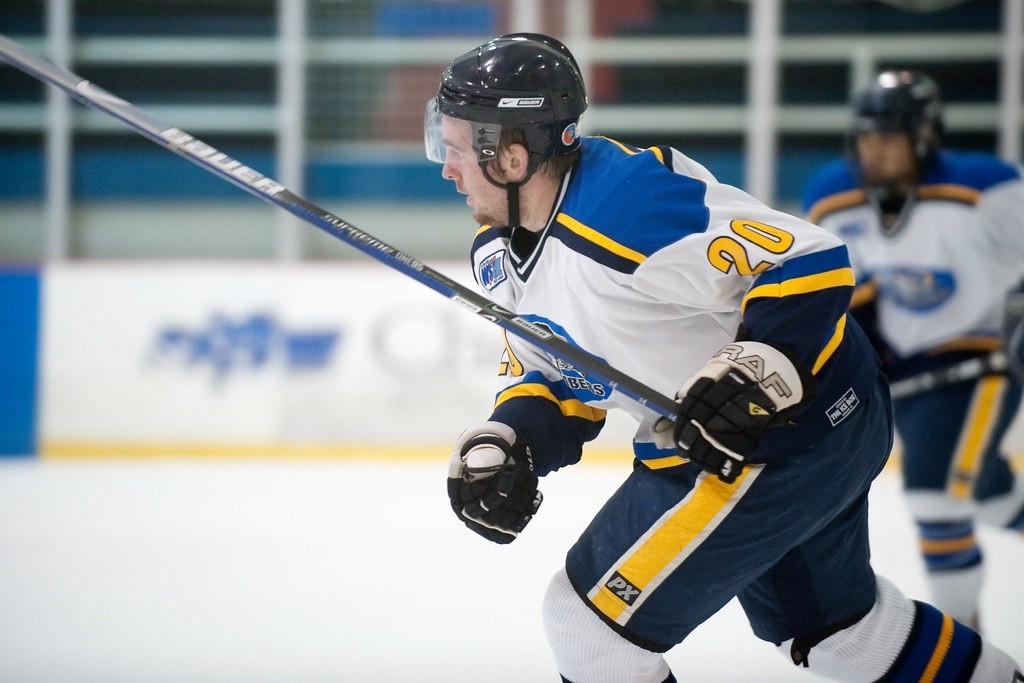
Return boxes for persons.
[421,33,1024,683]
[801,65,1024,632]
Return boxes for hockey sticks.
[0,37,682,422]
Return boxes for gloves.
[670,340,823,484]
[447,420,543,545]
[1004,279,1024,382]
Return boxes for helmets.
[849,71,948,166]
[435,32,587,126]
